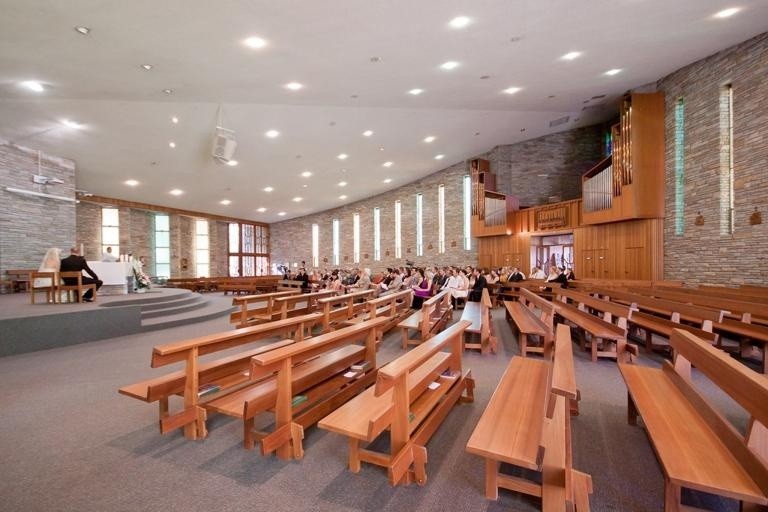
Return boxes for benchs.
[2,268,40,292]
[227,267,768,361]
[618,328,767,512]
[465,323,593,510]
[318,319,475,487]
[120,311,326,440]
[206,315,394,459]
[162,274,256,296]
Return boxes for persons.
[32,248,73,303]
[281,260,575,308]
[60,246,103,302]
[101,247,120,263]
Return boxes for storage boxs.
[2,268,40,292]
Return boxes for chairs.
[56,270,97,305]
[28,270,72,305]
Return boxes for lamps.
[56,270,97,305]
[28,270,72,305]
